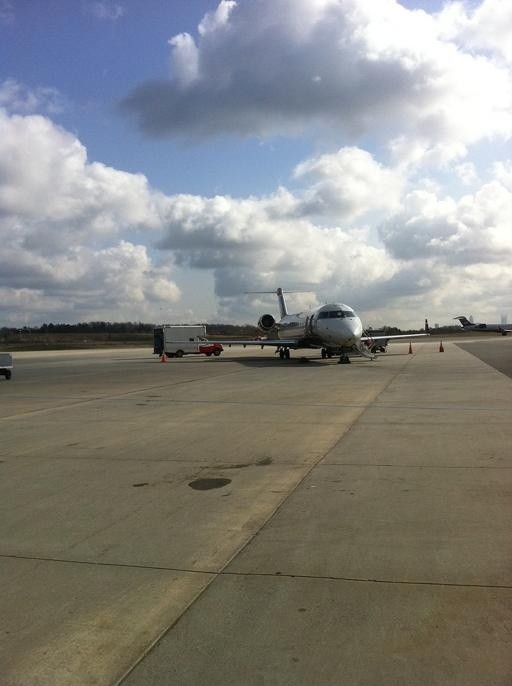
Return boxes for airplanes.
[453,314,511,336]
[163,286,431,364]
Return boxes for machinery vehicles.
[323,328,389,353]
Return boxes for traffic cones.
[409,340,413,354]
[161,350,168,363]
[439,340,444,352]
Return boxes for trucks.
[153,324,224,358]
[0,353,14,381]
